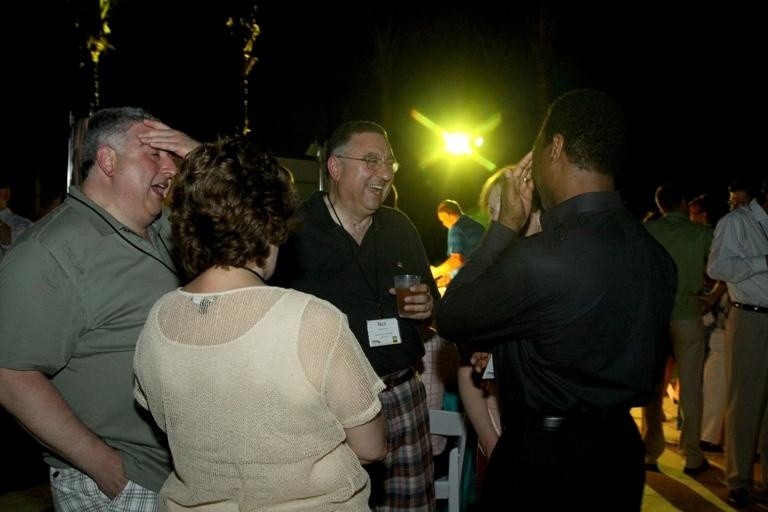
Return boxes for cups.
[394,274,421,318]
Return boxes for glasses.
[333,155,399,174]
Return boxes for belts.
[502,404,629,430]
[729,302,768,315]
[378,370,417,394]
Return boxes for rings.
[523,177,531,183]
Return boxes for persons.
[706,189,768,507]
[416,319,463,481]
[455,163,543,512]
[132,136,389,512]
[642,184,726,476]
[0,109,205,512]
[699,179,762,465]
[1,178,34,263]
[433,88,676,512]
[429,198,487,296]
[260,120,442,512]
[685,192,719,350]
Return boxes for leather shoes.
[727,487,752,505]
[648,463,659,469]
[697,439,723,453]
[684,457,710,473]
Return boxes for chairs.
[424,409,470,511]
